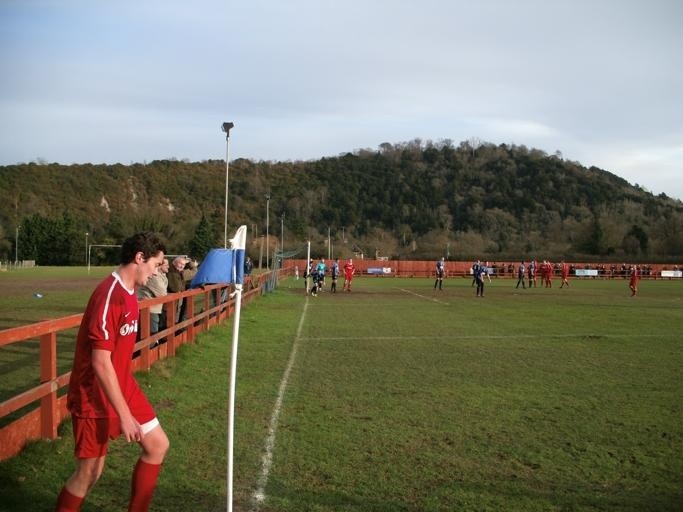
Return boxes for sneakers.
[311,285,352,297]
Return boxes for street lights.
[222,121,234,249]
[15,224,22,264]
[85,232,88,265]
[280,209,286,269]
[266,193,270,270]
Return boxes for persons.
[432,256,445,291]
[302,256,355,298]
[468,254,683,299]
[52,233,172,512]
[134,248,254,358]
